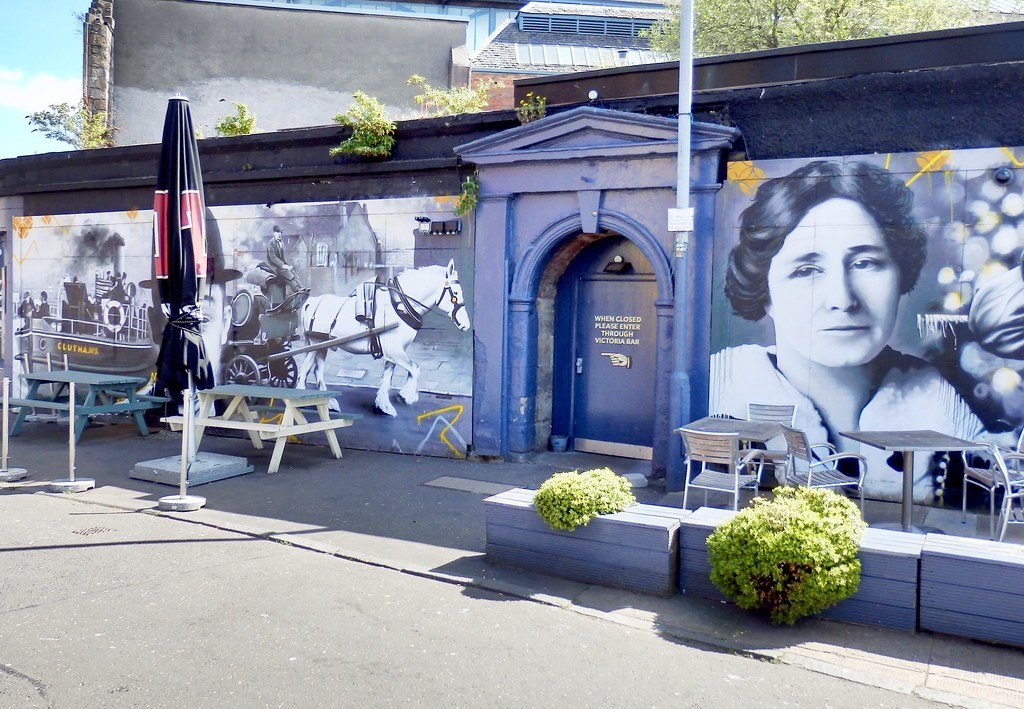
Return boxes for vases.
[549,435,567,451]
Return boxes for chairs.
[989,443,1024,542]
[962,428,1024,540]
[678,427,765,511]
[740,404,798,489]
[780,424,868,520]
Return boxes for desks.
[9,370,149,444]
[673,417,793,510]
[194,383,343,473]
[838,430,989,535]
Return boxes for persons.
[113,207,230,437]
[708,160,993,509]
[16,267,130,338]
[265,226,303,309]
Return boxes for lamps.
[602,261,631,274]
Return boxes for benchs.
[105,390,167,404]
[0,396,81,411]
[246,404,364,421]
[159,415,284,431]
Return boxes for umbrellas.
[151,93,216,513]
